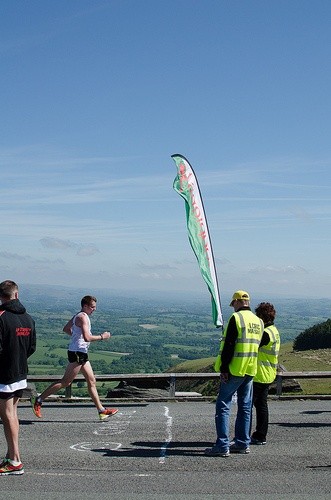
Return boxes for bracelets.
[100,334,103,340]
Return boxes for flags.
[173,154,225,329]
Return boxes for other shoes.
[204,447,230,456]
[229,443,251,453]
[250,437,267,444]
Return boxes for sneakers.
[0,460,25,475]
[29,396,43,418]
[98,408,118,419]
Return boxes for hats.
[229,290,250,306]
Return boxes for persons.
[0,280,36,475]
[229,302,280,446]
[31,295,118,420]
[205,290,264,456]
